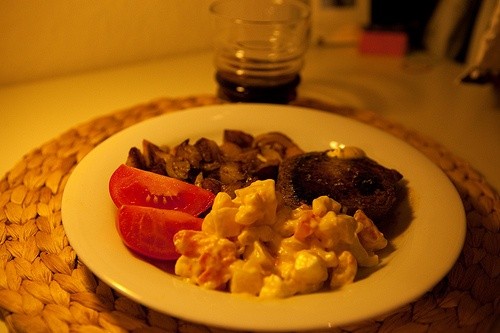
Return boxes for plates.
[62,103,467,331]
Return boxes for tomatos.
[116,205,206,262]
[109,164,216,217]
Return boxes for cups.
[208,1,310,103]
